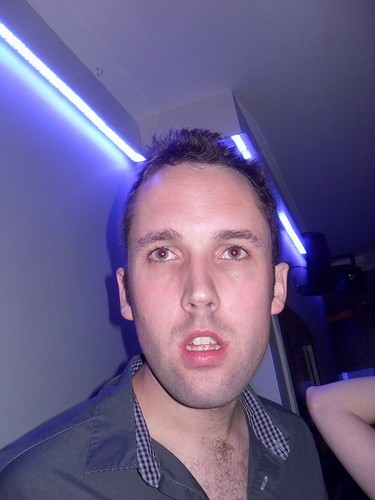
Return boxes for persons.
[0,126,327,500]
[305,376,375,500]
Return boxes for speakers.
[325,265,371,312]
